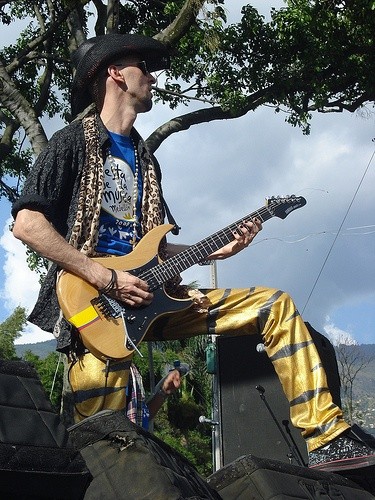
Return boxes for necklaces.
[105,134,138,252]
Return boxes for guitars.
[55,193,307,364]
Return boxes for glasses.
[106,61,148,75]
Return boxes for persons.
[12,35,374,474]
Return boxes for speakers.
[210,319,340,474]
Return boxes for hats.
[71,34,172,117]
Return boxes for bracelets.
[99,268,117,294]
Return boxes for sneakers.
[308,437,375,472]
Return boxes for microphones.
[199,416,220,425]
